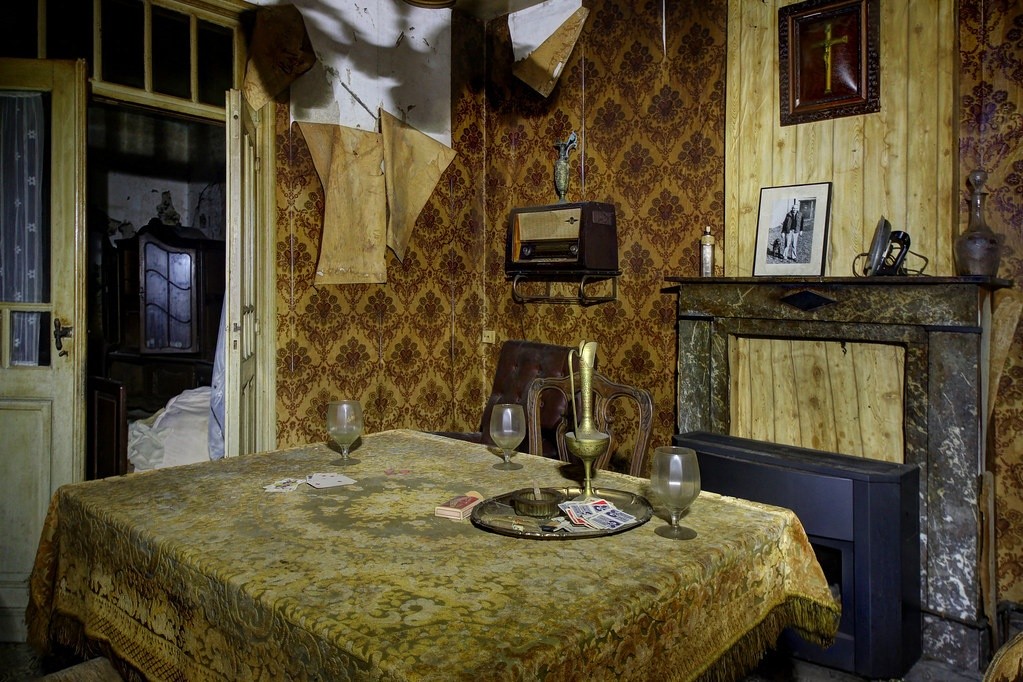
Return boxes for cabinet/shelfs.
[85,218,225,481]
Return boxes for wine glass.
[326,400,364,465]
[489,404,526,470]
[651,446,700,539]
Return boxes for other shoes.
[784,256,787,259]
[793,258,798,263]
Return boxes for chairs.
[420,340,599,467]
[527,370,656,477]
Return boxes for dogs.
[770,238,781,258]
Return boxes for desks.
[22,429,841,682]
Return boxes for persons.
[781,205,803,263]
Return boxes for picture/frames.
[778,0,880,127]
[752,182,832,277]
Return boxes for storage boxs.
[435,491,485,521]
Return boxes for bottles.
[700,230,715,278]
[953,170,1001,275]
